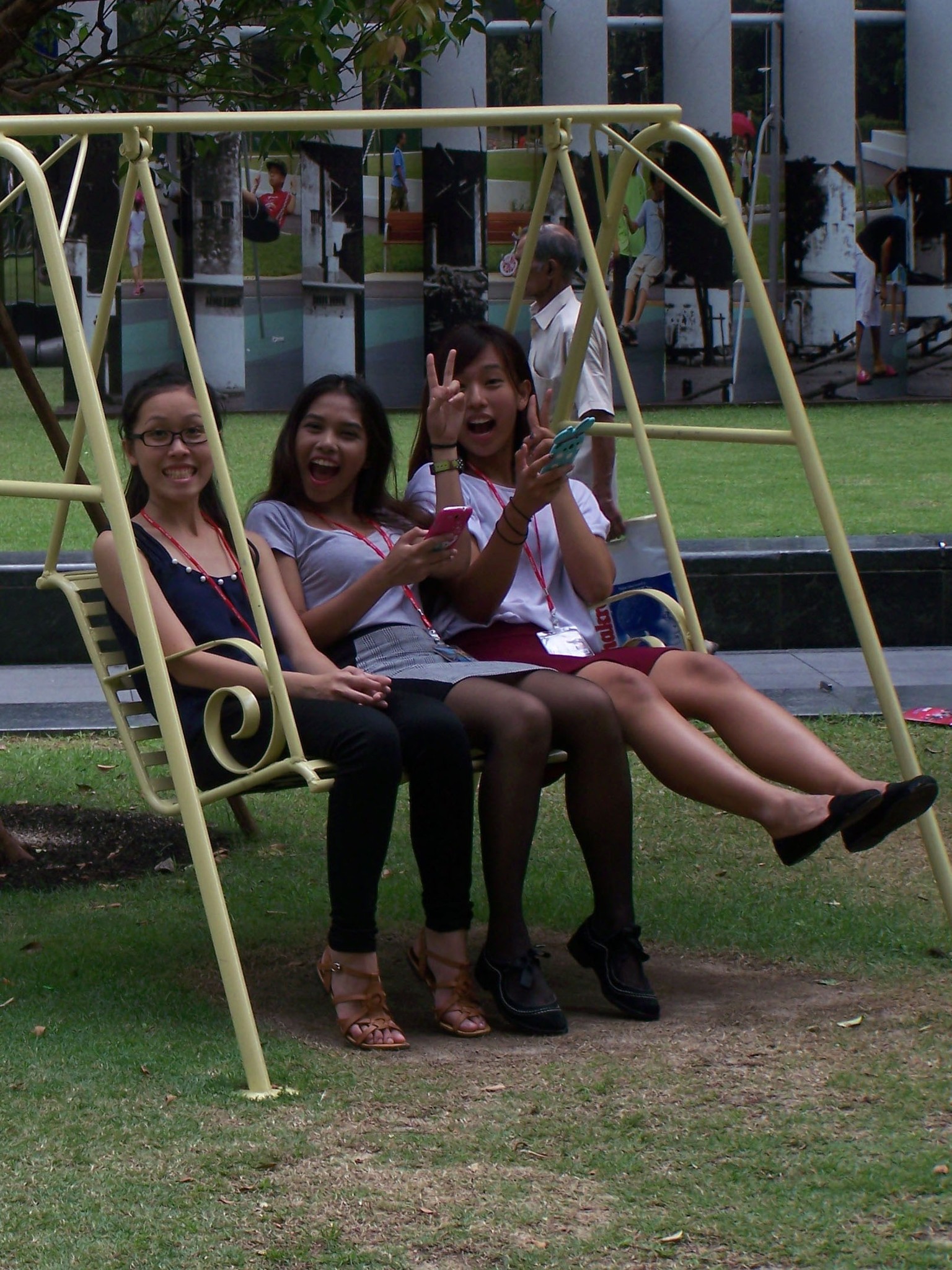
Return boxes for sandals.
[889,323,897,335]
[309,951,411,1051]
[899,322,907,333]
[406,927,491,1038]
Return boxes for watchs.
[429,459,463,475]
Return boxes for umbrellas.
[733,112,757,154]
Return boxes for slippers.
[872,364,898,379]
[856,369,870,383]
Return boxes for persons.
[172,159,297,241]
[884,167,924,336]
[734,133,752,205]
[243,350,662,1035]
[402,322,939,863]
[515,224,626,541]
[389,132,410,212]
[94,363,490,1048]
[855,215,933,384]
[125,192,147,296]
[617,169,664,344]
[610,159,646,326]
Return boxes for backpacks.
[746,149,756,182]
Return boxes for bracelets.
[292,192,296,196]
[496,521,527,545]
[510,497,533,521]
[430,443,457,449]
[503,507,528,536]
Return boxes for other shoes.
[473,931,568,1036]
[840,775,939,855]
[567,914,661,1021]
[618,324,637,347]
[139,284,146,294]
[133,289,141,296]
[607,517,626,544]
[772,788,881,867]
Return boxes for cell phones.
[424,505,473,551]
[541,417,595,475]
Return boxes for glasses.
[129,425,208,447]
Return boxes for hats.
[265,158,288,177]
[135,190,144,203]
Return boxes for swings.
[32,128,720,812]
[241,127,297,247]
[621,120,670,294]
